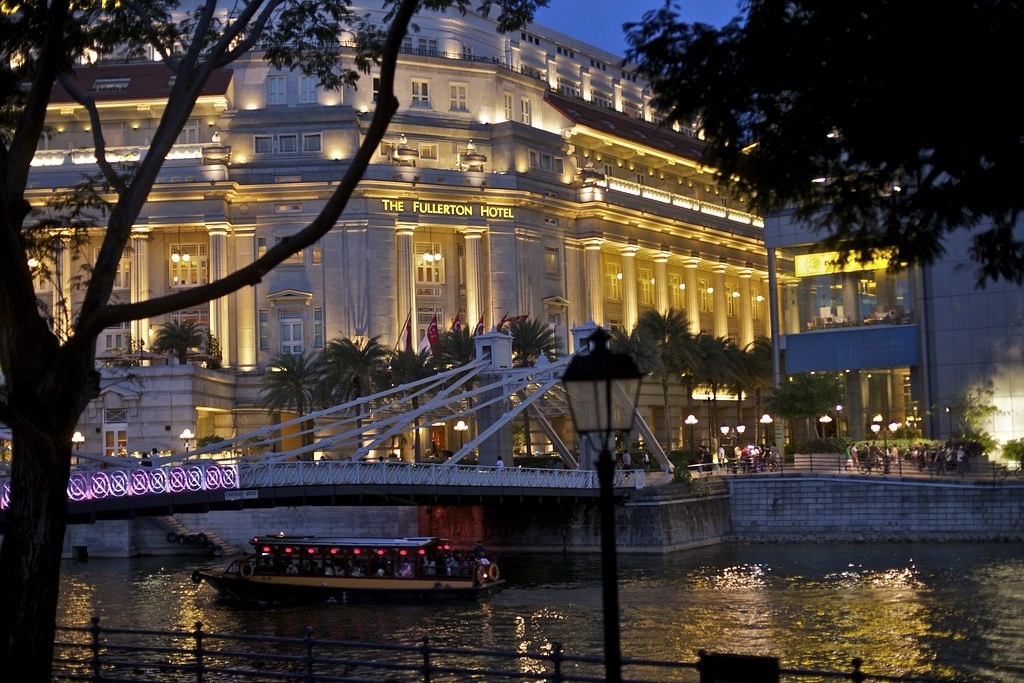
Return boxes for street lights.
[759,413,773,456]
[869,414,900,474]
[453,419,469,464]
[179,428,195,460]
[560,327,648,683]
[72,431,86,468]
[685,414,699,468]
[719,421,745,445]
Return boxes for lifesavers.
[488,563,500,580]
[192,571,201,583]
[239,562,254,580]
[476,566,487,586]
[167,531,224,557]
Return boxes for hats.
[959,446,963,449]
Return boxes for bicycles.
[757,453,783,472]
[996,463,1024,481]
[857,456,887,476]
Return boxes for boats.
[191,535,508,608]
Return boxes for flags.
[474,317,483,336]
[497,316,505,331]
[506,315,528,324]
[420,315,439,356]
[448,314,462,338]
[402,316,412,353]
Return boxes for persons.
[622,450,631,475]
[845,443,859,469]
[913,441,966,476]
[698,442,780,472]
[863,441,898,474]
[296,453,403,468]
[642,450,650,471]
[282,540,490,580]
[141,448,161,467]
[496,456,504,472]
